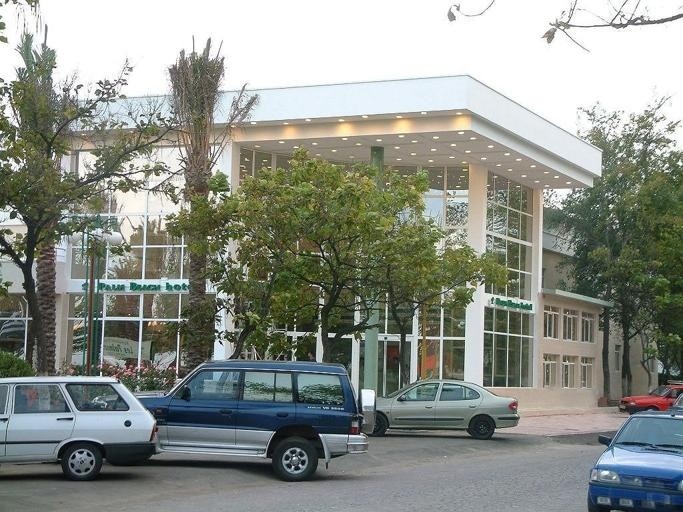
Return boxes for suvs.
[88,357,380,484]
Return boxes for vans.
[0,374,167,484]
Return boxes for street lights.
[80,228,125,373]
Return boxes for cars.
[366,375,521,442]
[585,380,683,512]
